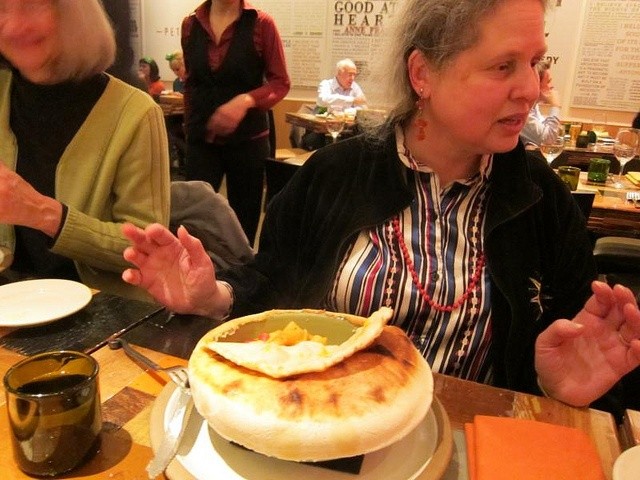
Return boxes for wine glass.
[593,114,607,152]
[325,107,348,145]
[610,129,639,190]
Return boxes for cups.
[556,166,581,191]
[570,120,582,143]
[3,350,103,476]
[585,157,611,186]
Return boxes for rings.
[618,334,629,346]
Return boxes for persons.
[121,0,639,409]
[1,0,172,279]
[520,58,561,152]
[136,56,166,101]
[302,57,369,149]
[166,50,190,159]
[181,0,292,252]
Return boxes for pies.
[187,305,434,463]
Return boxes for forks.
[626,192,640,212]
[106,336,190,395]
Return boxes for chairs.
[169,181,253,272]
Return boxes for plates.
[149,378,457,476]
[0,278,93,326]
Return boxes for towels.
[465,415,606,480]
[625,172,640,186]
[580,130,609,137]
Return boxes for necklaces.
[392,214,486,312]
